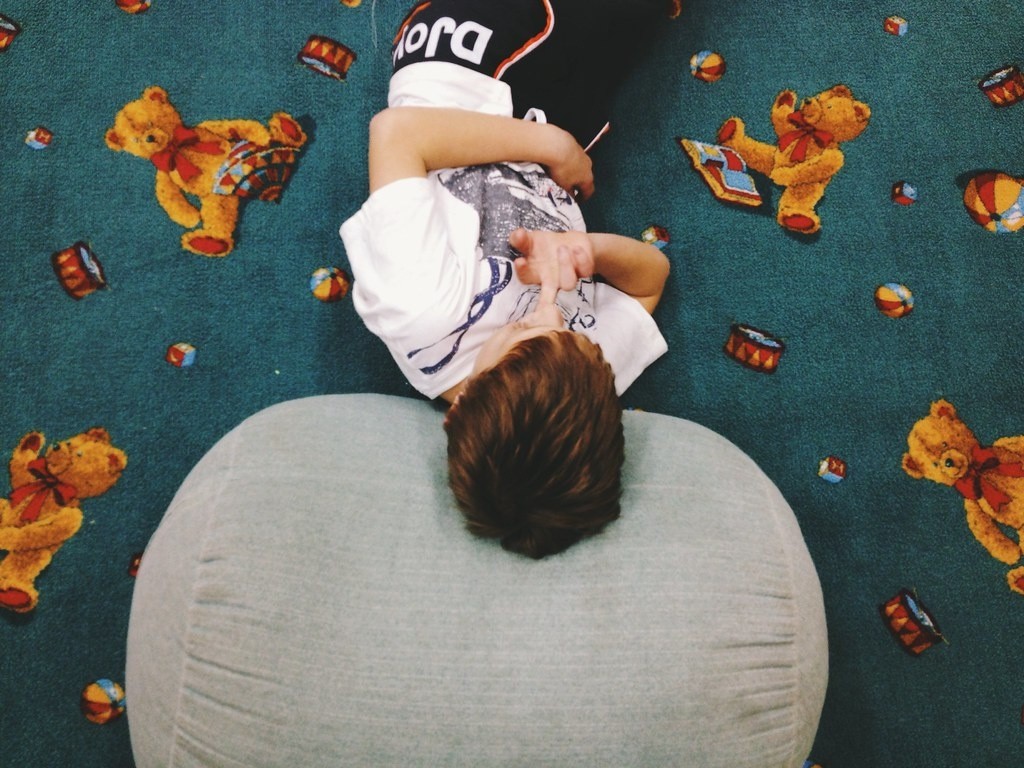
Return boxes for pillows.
[125,391,829,767]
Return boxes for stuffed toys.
[1,428,128,613]
[901,399,1024,594]
[106,86,306,259]
[718,84,871,235]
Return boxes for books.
[680,135,762,208]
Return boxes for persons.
[341,0,671,558]
